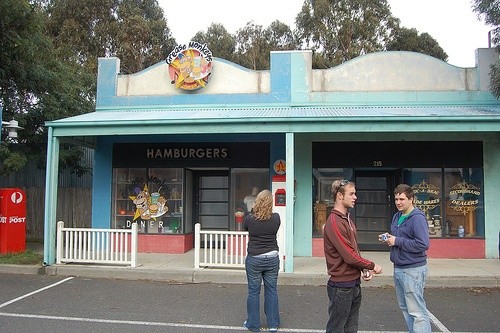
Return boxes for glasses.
[335,180,349,194]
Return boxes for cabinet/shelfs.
[115,168,183,235]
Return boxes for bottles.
[458,225,464,237]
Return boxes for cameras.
[381,234,387,240]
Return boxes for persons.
[323,179,383,333]
[244,185,260,213]
[378,184,432,333]
[243,189,281,332]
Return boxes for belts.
[251,254,277,258]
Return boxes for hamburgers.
[150,205,158,215]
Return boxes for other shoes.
[244,320,260,331]
